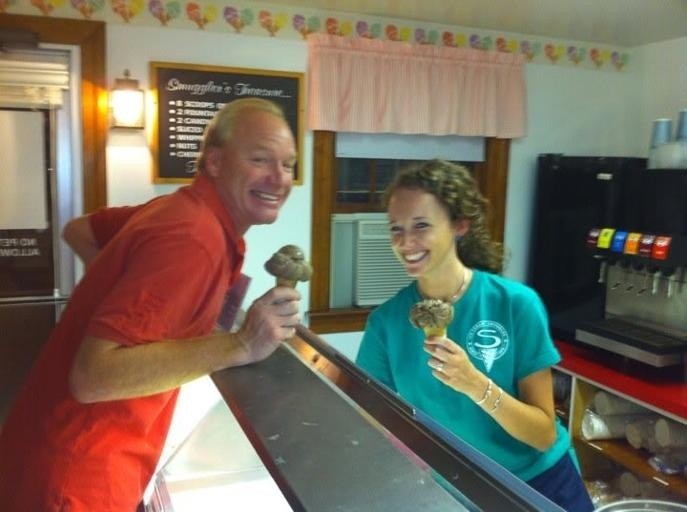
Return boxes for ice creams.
[473,328,502,375]
[408,299,455,337]
[265,245,314,288]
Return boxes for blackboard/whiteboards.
[149,61,306,186]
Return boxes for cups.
[619,471,642,498]
[581,389,687,451]
[643,110,686,169]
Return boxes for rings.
[435,361,445,372]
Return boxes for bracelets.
[487,386,504,415]
[476,378,492,407]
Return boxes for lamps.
[105,65,148,131]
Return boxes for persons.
[3,97,301,512]
[355,158,595,512]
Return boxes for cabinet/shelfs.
[142,305,568,512]
[545,363,687,506]
